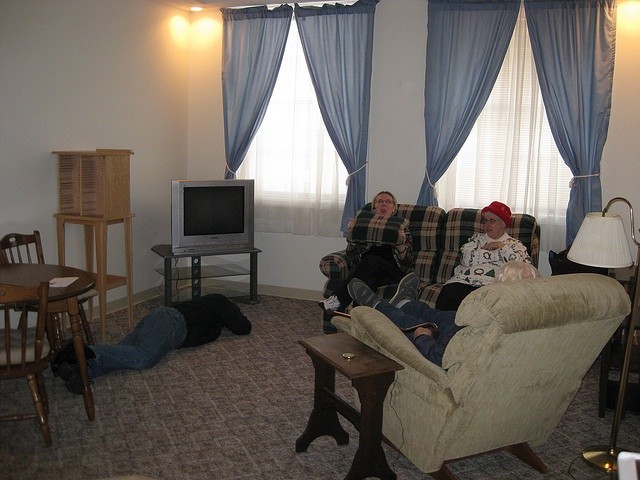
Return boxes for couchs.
[331,274,632,480]
[317,202,541,336]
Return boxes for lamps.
[566,198,639,472]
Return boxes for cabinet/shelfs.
[53,147,136,340]
[598,279,640,421]
[151,242,261,307]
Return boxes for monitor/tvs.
[171,179,256,255]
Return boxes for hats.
[481,201,512,227]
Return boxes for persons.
[347,260,542,367]
[435,200,531,310]
[48,293,252,394]
[317,191,415,316]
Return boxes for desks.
[1,262,97,421]
[296,331,404,480]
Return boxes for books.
[49,276,79,288]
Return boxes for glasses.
[481,218,500,224]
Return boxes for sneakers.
[347,277,382,309]
[318,295,341,316]
[389,272,420,306]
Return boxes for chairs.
[0,280,55,447]
[2,231,99,354]
